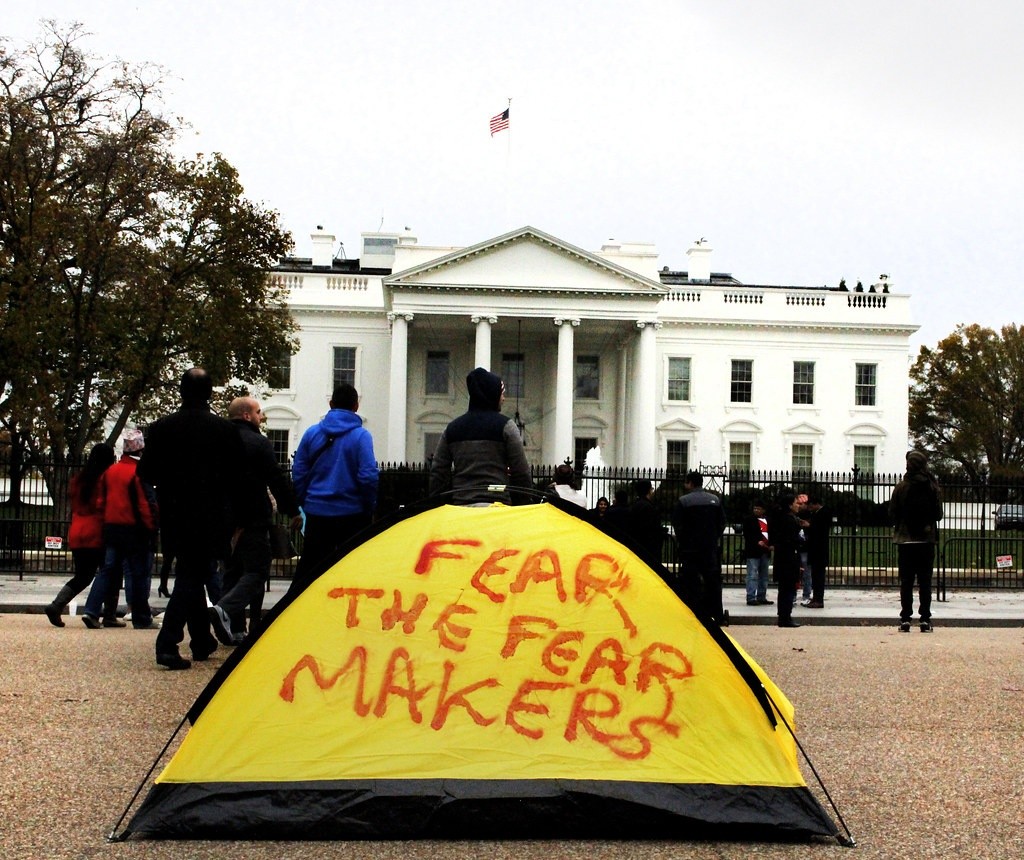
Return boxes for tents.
[108,485,855,847]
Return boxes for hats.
[122,430,145,452]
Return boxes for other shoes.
[919,621,934,632]
[45,605,66,627]
[800,597,812,606]
[123,612,133,621]
[760,598,775,604]
[82,612,101,628]
[101,616,126,628]
[898,621,910,633]
[234,630,248,641]
[134,618,163,629]
[748,599,762,606]
[803,601,824,608]
[156,652,192,670]
[777,619,799,628]
[208,602,233,646]
[193,639,219,661]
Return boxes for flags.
[489,108,509,135]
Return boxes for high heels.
[158,585,172,598]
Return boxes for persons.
[429,368,533,504]
[546,464,587,508]
[590,497,610,516]
[207,397,304,646]
[291,385,379,554]
[888,451,943,632]
[44,429,162,628]
[602,481,669,563]
[743,493,828,627]
[671,473,723,612]
[137,369,252,670]
